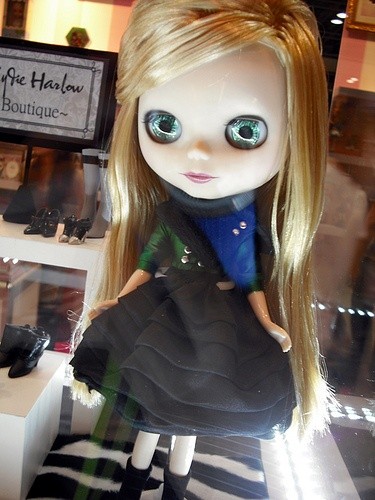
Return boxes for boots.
[86,153,112,239]
[76,149,106,227]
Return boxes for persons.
[69,1,330,500]
[307,96,375,399]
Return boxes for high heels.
[24,210,47,234]
[59,215,76,242]
[43,209,59,237]
[0,324,31,368]
[68,218,90,245]
[8,329,49,378]
